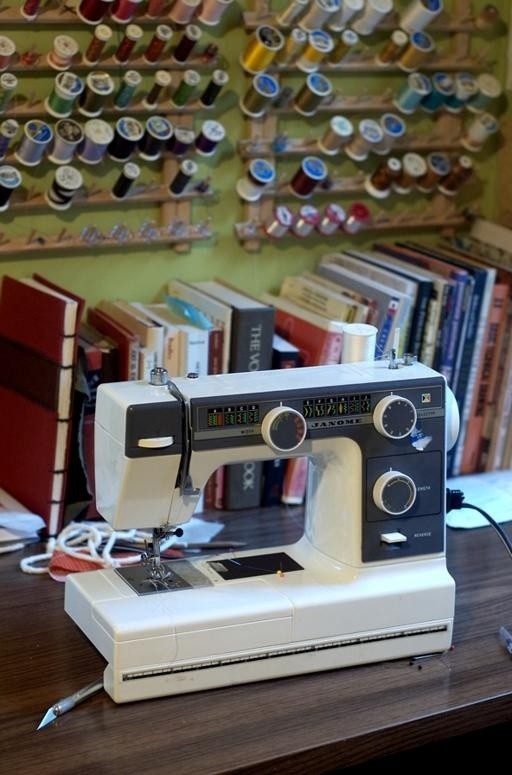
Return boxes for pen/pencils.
[173,540,246,551]
[497,626,512,654]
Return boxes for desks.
[3,462,508,774]
